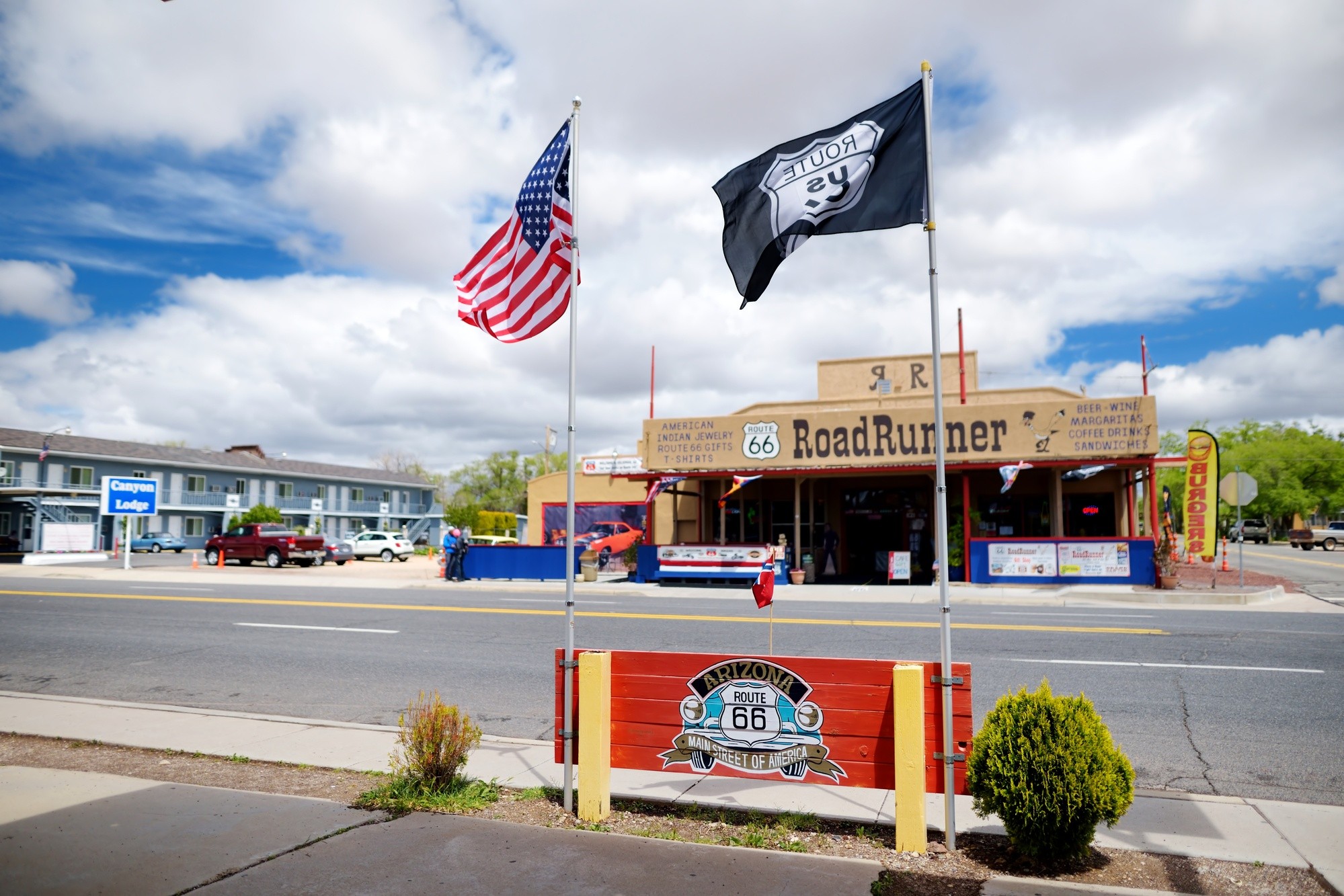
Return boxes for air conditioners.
[376,496,384,501]
[226,485,237,493]
[210,484,221,492]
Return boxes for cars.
[123,532,188,553]
[468,535,518,545]
[311,535,353,566]
[555,521,645,566]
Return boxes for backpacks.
[458,538,469,554]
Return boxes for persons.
[443,525,468,582]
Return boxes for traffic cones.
[218,550,225,568]
[191,553,199,569]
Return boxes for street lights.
[33,426,72,553]
[532,439,550,475]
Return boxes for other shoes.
[454,578,464,582]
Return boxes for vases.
[790,571,806,585]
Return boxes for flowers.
[791,568,805,572]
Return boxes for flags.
[1062,464,1116,480]
[452,116,581,343]
[39,436,50,461]
[999,460,1034,494]
[718,475,764,508]
[752,555,775,610]
[711,80,929,308]
[644,476,687,504]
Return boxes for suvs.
[342,530,414,562]
[1228,519,1270,544]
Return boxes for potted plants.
[1158,561,1179,590]
[623,537,643,578]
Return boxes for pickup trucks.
[1288,520,1344,551]
[205,522,324,568]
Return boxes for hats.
[448,526,454,532]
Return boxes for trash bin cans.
[579,549,599,581]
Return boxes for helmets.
[453,529,461,537]
